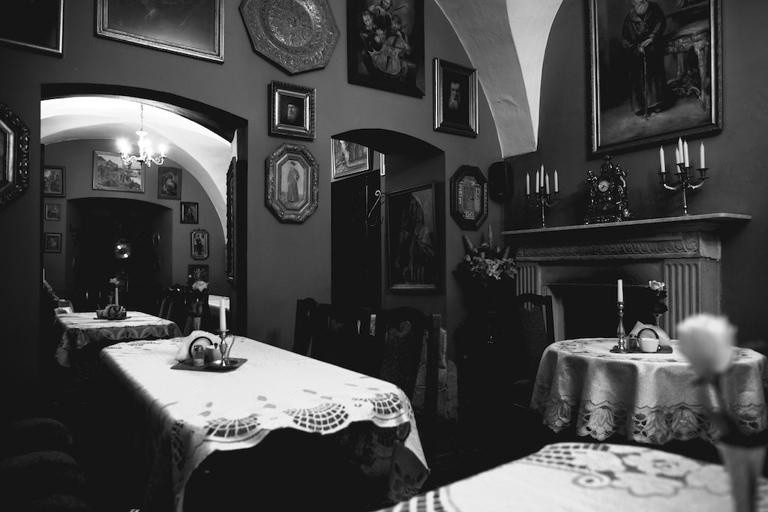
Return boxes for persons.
[444,81,468,121]
[355,0,415,76]
[281,101,304,127]
[622,0,667,116]
[399,194,432,285]
[287,161,299,202]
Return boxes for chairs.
[156,286,555,421]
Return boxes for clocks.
[582,153,632,225]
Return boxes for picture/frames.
[237,0,338,75]
[332,139,373,178]
[267,79,316,141]
[450,166,488,230]
[346,2,425,99]
[265,143,319,224]
[381,179,442,296]
[584,0,723,165]
[92,0,227,65]
[42,144,211,287]
[432,57,479,138]
[0,104,30,208]
[0,0,68,59]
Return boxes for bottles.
[193,345,205,367]
[121,308,126,317]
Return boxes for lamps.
[113,101,171,177]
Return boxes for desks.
[55,302,767,511]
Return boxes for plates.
[637,328,659,344]
[190,337,213,358]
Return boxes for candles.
[524,162,559,195]
[657,135,707,173]
[617,279,624,302]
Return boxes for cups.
[639,338,660,353]
[206,345,221,362]
[96,310,103,319]
[627,339,637,352]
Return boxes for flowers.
[456,229,519,285]
